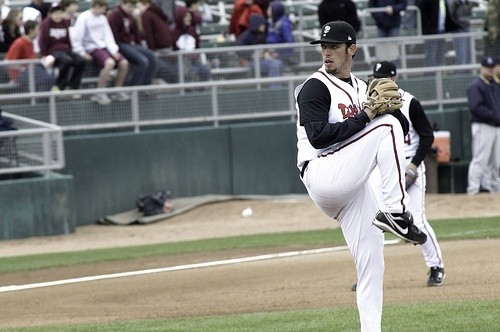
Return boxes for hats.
[481,56,497,67]
[368,62,396,78]
[310,22,356,44]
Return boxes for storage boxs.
[437,161,470,194]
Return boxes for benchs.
[0,0,488,90]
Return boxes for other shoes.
[91,92,110,104]
[112,92,129,101]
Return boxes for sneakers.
[373,210,427,244]
[428,266,445,286]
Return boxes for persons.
[69,0,131,105]
[0,0,212,92]
[413,0,462,77]
[368,0,408,79]
[227,0,264,79]
[3,20,55,106]
[351,60,447,291]
[106,0,159,96]
[448,0,473,74]
[318,0,362,36]
[38,2,85,101]
[234,13,284,88]
[135,0,176,53]
[466,57,500,194]
[293,21,428,332]
[266,2,300,75]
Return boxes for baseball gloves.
[363,76,404,116]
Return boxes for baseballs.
[241,208,253,218]
